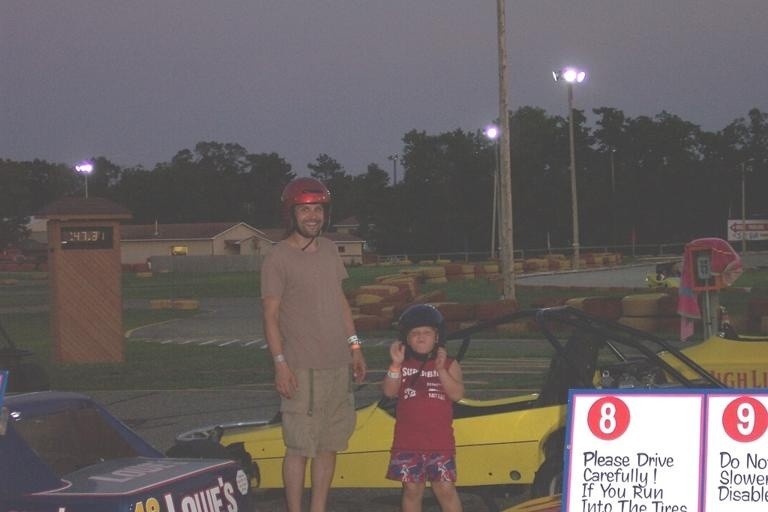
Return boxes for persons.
[260,175,370,512]
[380,303,465,512]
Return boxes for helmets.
[280,177,332,233]
[397,304,445,347]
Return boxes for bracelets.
[348,335,358,344]
[351,341,362,345]
[272,353,286,365]
[387,371,401,379]
[351,344,360,349]
[388,366,401,372]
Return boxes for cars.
[653,309,768,390]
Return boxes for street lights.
[739,158,756,255]
[486,124,502,259]
[552,65,586,269]
[388,152,401,186]
[75,160,95,197]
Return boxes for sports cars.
[167,306,729,512]
[1,389,251,512]
[643,261,682,288]
